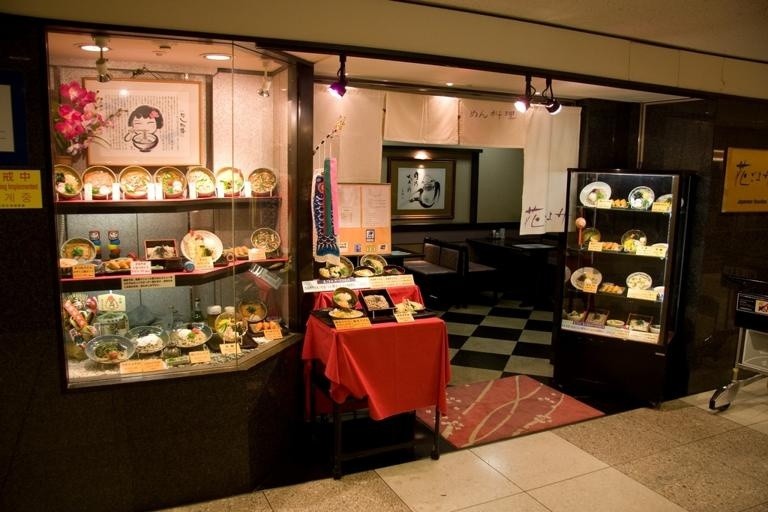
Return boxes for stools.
[408,244,464,309]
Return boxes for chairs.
[460,244,499,305]
[403,237,441,268]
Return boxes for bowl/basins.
[606,319,625,328]
[649,324,661,334]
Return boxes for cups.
[206,305,223,334]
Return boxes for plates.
[53,163,83,198]
[611,202,630,210]
[656,192,684,211]
[186,166,217,198]
[250,227,281,253]
[332,287,360,311]
[249,321,284,333]
[602,244,624,253]
[237,298,268,324]
[396,304,426,311]
[214,167,245,196]
[81,165,119,199]
[352,266,376,277]
[153,166,187,198]
[599,285,627,295]
[143,238,179,266]
[625,313,653,333]
[83,334,137,365]
[628,185,655,210]
[214,312,249,342]
[118,166,153,199]
[359,253,388,276]
[579,181,612,208]
[59,236,98,262]
[247,167,279,196]
[180,229,223,264]
[125,323,171,355]
[581,227,601,246]
[358,288,397,316]
[583,306,610,326]
[235,248,251,260]
[168,323,214,350]
[570,266,602,293]
[316,252,354,281]
[621,229,647,252]
[382,265,406,275]
[625,271,652,290]
[328,308,365,320]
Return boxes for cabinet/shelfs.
[551,167,698,347]
[56,194,301,391]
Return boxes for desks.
[467,238,557,295]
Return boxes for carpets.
[413,373,606,448]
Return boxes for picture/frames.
[387,156,457,221]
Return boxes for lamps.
[514,75,562,116]
[91,34,114,83]
[256,55,272,99]
[328,55,346,98]
